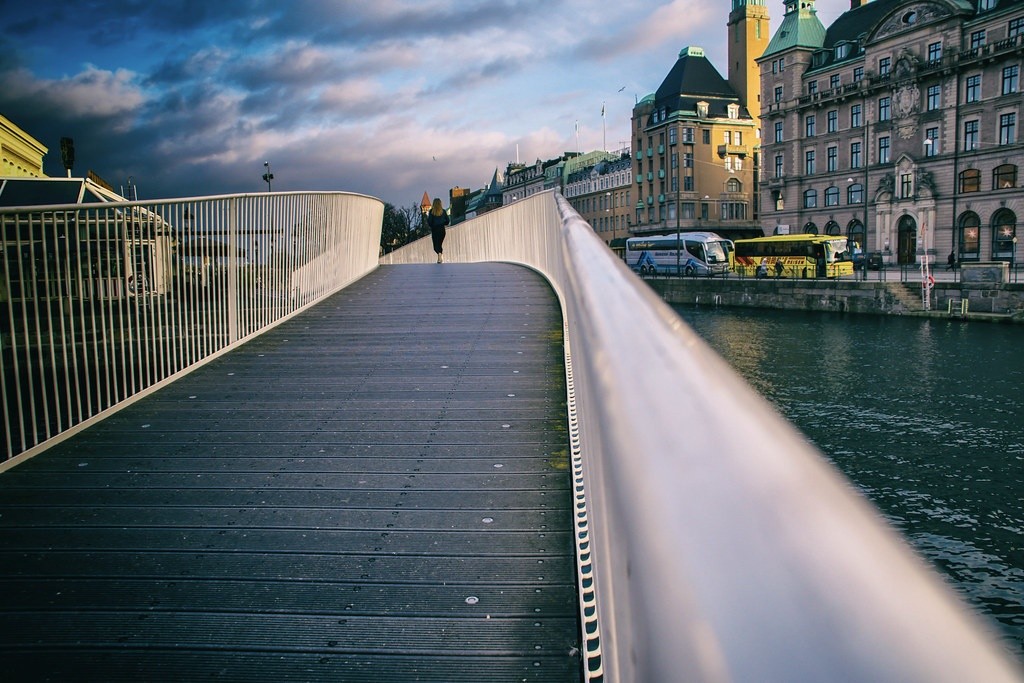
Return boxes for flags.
[601,105,604,115]
[574,121,579,133]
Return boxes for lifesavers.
[923,275,935,289]
[128,274,150,295]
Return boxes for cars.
[852,252,883,272]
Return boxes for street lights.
[605,189,616,239]
[261,161,274,242]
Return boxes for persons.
[946,251,956,273]
[428,198,450,263]
[774,258,784,281]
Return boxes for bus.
[626,232,734,278]
[727,234,854,278]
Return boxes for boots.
[437,253,443,263]
